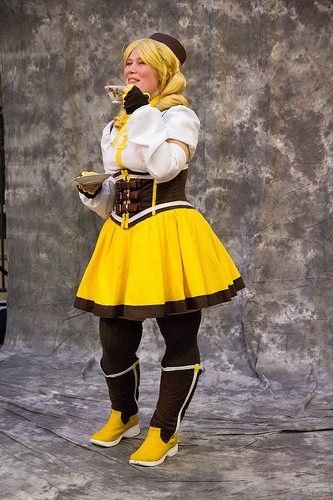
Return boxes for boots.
[90,357,140,448]
[128,363,205,468]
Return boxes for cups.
[104,86,127,103]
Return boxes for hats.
[149,32,186,65]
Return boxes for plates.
[75,173,111,185]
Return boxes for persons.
[73,32,246,466]
